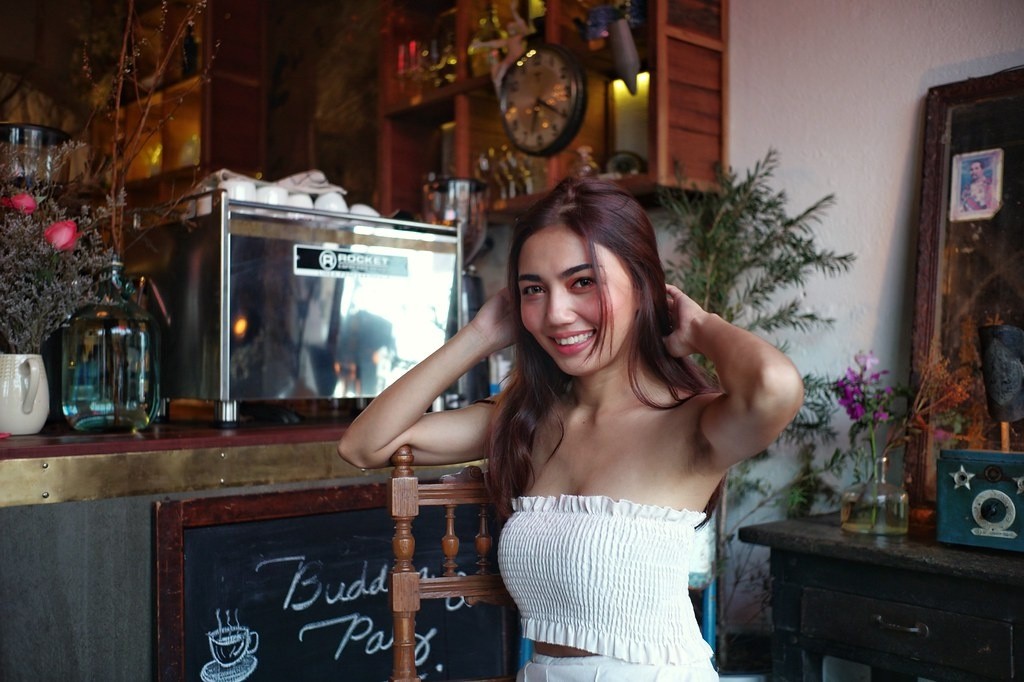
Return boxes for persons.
[336,175,805,682]
[961,159,996,211]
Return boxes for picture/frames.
[948,145,1003,222]
[905,65,1024,523]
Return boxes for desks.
[1,418,489,681]
[737,510,1023,682]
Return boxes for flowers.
[0,136,135,354]
[833,350,982,525]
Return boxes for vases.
[60,259,161,432]
[0,355,50,435]
[839,456,909,535]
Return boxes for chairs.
[389,444,523,682]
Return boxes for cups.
[184,178,383,228]
[1,353,51,436]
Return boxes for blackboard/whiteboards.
[151,474,521,682]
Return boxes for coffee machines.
[420,174,493,414]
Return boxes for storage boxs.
[935,449,1024,552]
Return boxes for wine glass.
[474,141,539,202]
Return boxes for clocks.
[497,41,587,158]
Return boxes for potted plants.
[660,148,845,682]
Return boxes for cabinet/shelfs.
[380,1,731,224]
[91,0,267,251]
